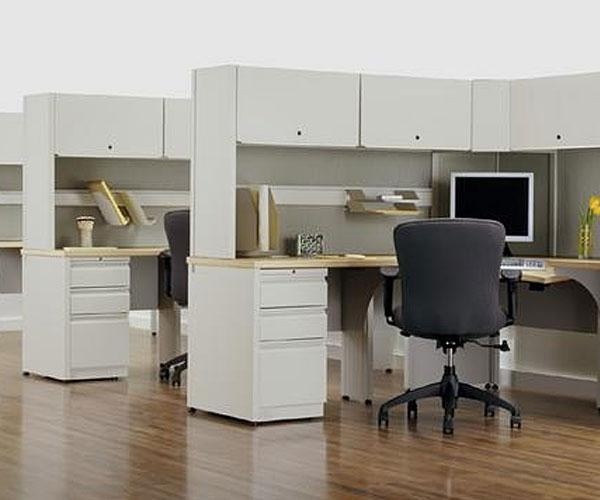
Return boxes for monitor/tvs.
[450,171,534,257]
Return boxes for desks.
[183,256,600,429]
[17,244,180,388]
[0,242,24,250]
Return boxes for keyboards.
[500,257,545,271]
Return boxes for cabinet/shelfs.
[509,74,599,155]
[193,66,359,150]
[361,73,509,154]
[163,97,191,159]
[1,111,27,165]
[24,89,164,160]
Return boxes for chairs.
[378,217,527,442]
[155,208,190,388]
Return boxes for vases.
[580,227,591,260]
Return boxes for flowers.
[576,191,599,226]
